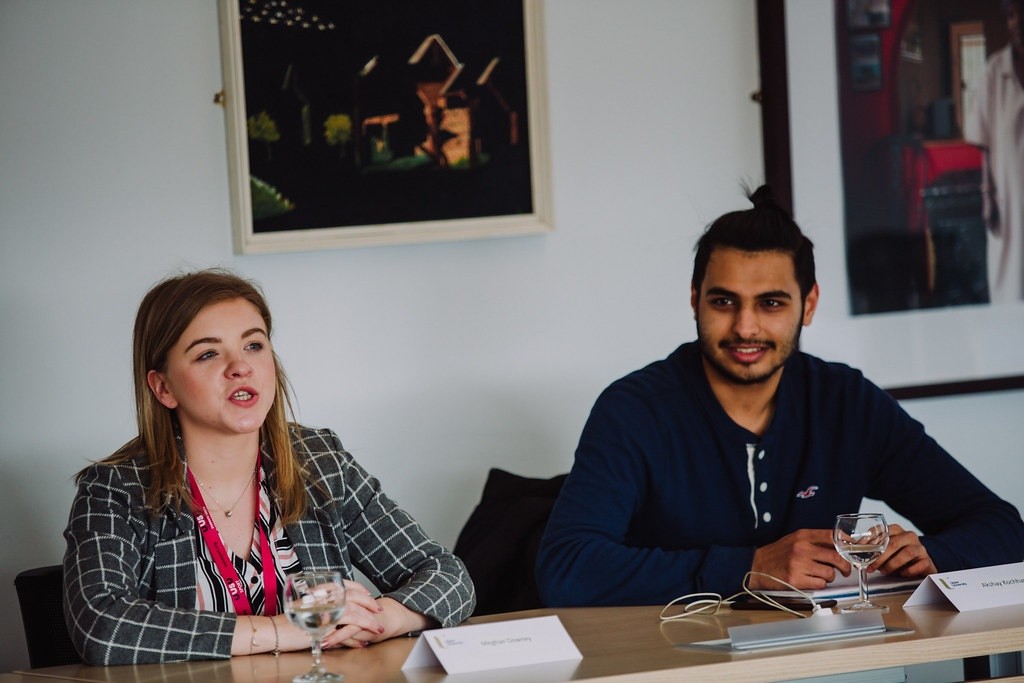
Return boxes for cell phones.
[729,596,838,610]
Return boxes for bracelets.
[268,615,281,655]
[247,614,259,655]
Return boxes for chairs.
[452,468,579,618]
[17,563,82,668]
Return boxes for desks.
[3,577,1024,681]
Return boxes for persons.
[532,185,1022,605]
[965,4,1024,301]
[59,267,479,664]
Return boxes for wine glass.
[283,572,347,682]
[833,513,890,613]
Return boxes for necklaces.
[184,461,264,518]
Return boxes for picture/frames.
[216,1,557,258]
[756,1,1022,402]
